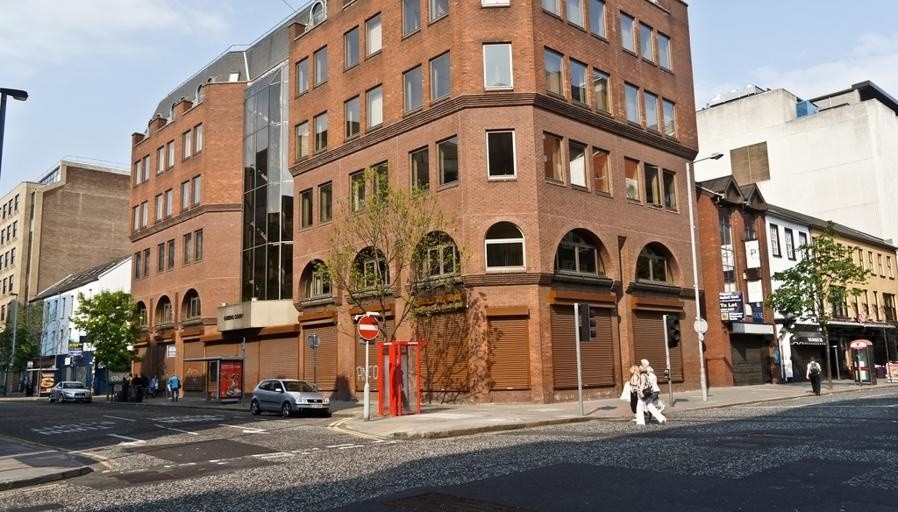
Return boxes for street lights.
[684,153,723,397]
[0,86,28,183]
[5,292,19,367]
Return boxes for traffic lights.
[578,303,596,340]
[665,315,678,349]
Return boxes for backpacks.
[809,362,820,378]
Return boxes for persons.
[112,371,182,403]
[621,359,666,426]
[803,356,822,396]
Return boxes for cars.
[49,381,91,403]
[250,377,329,416]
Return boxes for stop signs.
[358,316,380,340]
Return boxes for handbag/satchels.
[639,387,653,401]
